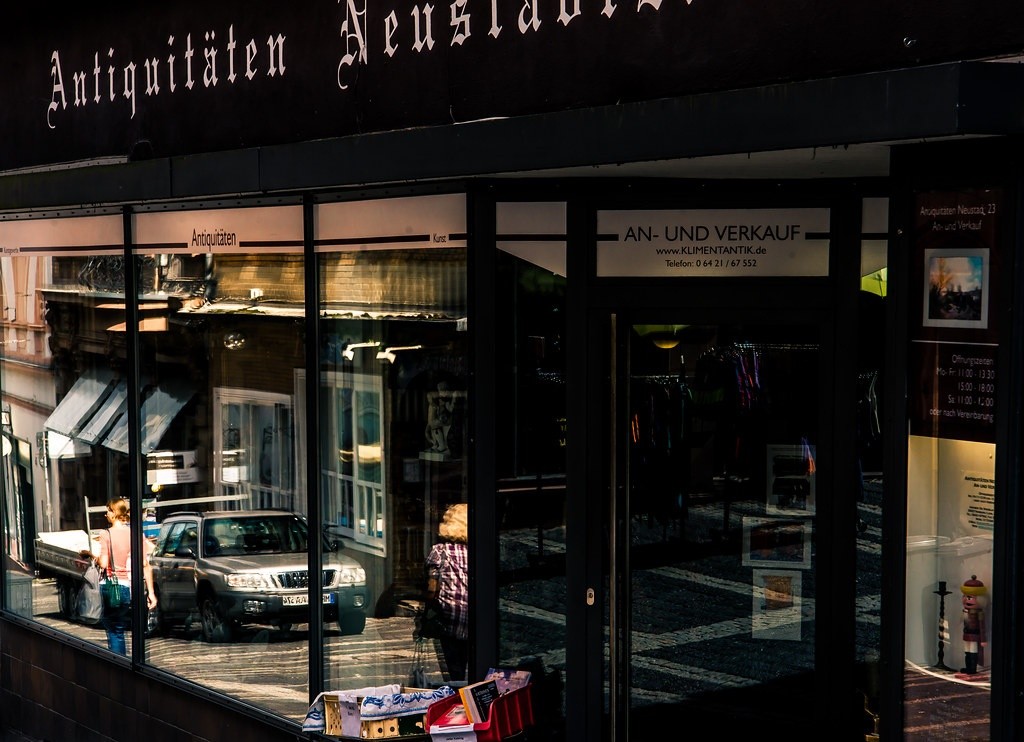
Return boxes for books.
[429,669,531,724]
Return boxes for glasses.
[106,510,110,515]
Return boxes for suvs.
[143,511,368,643]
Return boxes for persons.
[413,502,468,682]
[75,498,158,657]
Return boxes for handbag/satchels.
[79,558,102,620]
[99,584,130,633]
[412,598,450,640]
